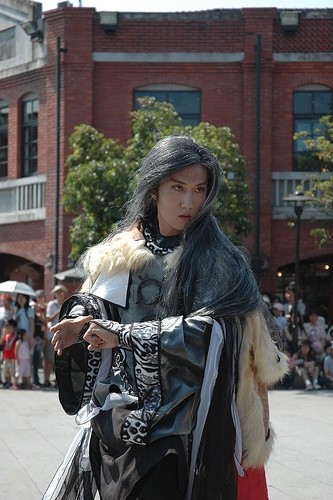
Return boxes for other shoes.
[1,379,59,390]
[306,384,313,390]
[313,384,321,390]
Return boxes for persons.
[0,285,69,389]
[260,284,333,392]
[39,135,289,500]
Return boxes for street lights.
[281,190,315,353]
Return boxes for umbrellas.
[0,280,36,298]
